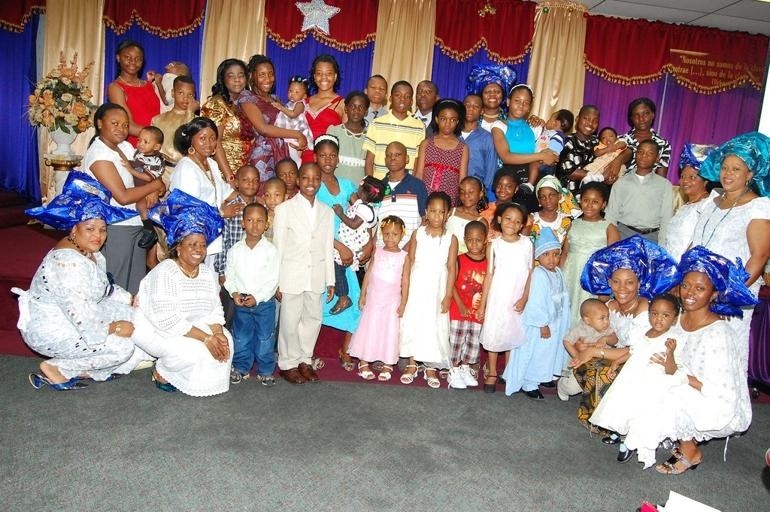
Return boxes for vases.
[50,125,77,155]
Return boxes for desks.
[42,153,84,230]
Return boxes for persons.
[346,64,770,475]
[16,38,388,398]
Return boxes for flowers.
[20,51,99,138]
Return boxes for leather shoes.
[522,382,556,401]
[329,295,353,316]
[601,433,634,463]
[484,372,499,393]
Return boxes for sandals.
[343,357,393,383]
[398,361,440,388]
[654,450,704,477]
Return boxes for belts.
[627,225,661,236]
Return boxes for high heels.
[27,370,87,393]
[281,358,326,384]
[149,366,176,392]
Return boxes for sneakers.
[441,362,479,390]
[230,369,276,386]
